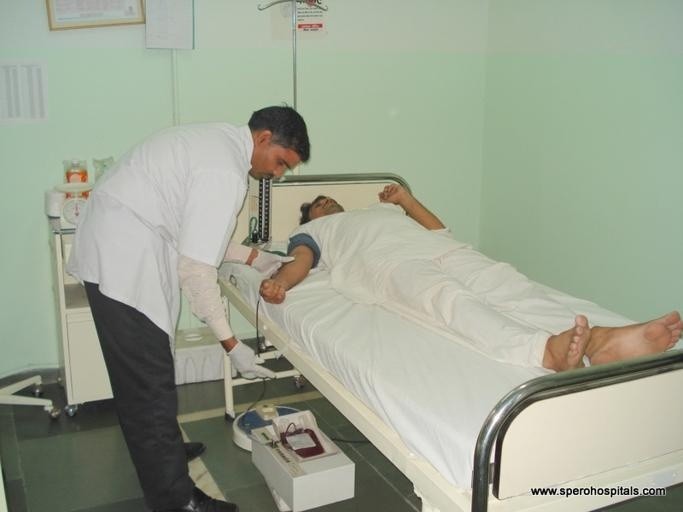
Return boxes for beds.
[217,172,682,512]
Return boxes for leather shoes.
[184,438,205,461]
[179,485,239,510]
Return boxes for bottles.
[66,159,89,201]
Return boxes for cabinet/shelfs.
[47,216,115,416]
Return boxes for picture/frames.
[45,0,146,30]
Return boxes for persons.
[66,105,312,511]
[259,182,683,375]
